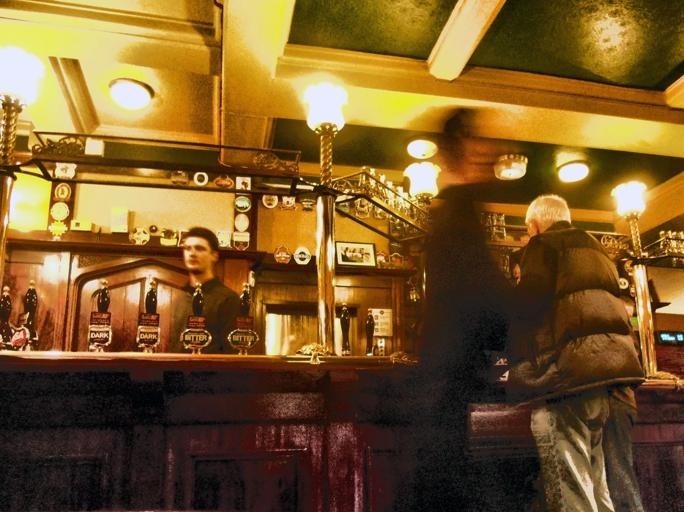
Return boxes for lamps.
[404,163,441,209]
[610,181,659,376]
[404,135,439,160]
[552,149,590,183]
[494,154,528,181]
[301,81,348,355]
[0,46,44,257]
[108,79,154,109]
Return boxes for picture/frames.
[335,240,377,268]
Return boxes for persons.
[398,103,551,510]
[500,193,649,511]
[161,223,242,355]
